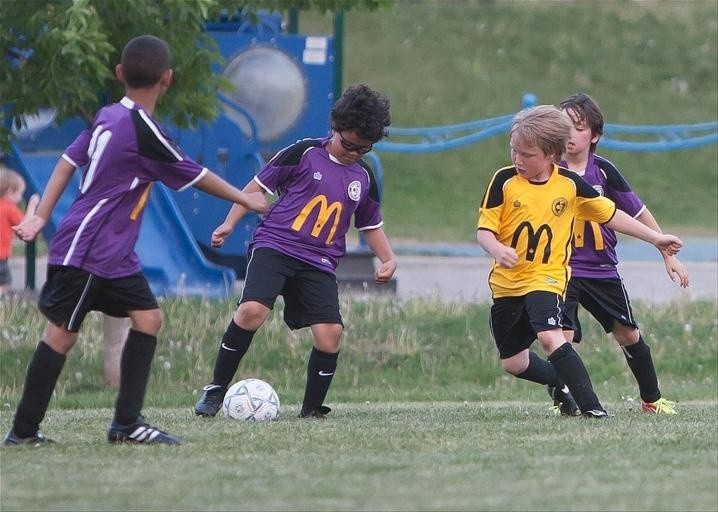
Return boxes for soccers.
[223,377,280,423]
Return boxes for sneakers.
[108,416,181,447]
[194,384,226,418]
[3,429,56,446]
[547,384,580,416]
[584,410,607,420]
[297,406,331,420]
[641,398,677,415]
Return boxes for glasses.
[339,130,372,154]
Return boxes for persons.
[194,82,398,419]
[3,35,269,451]
[546,93,689,412]
[0,169,38,290]
[477,104,682,419]
